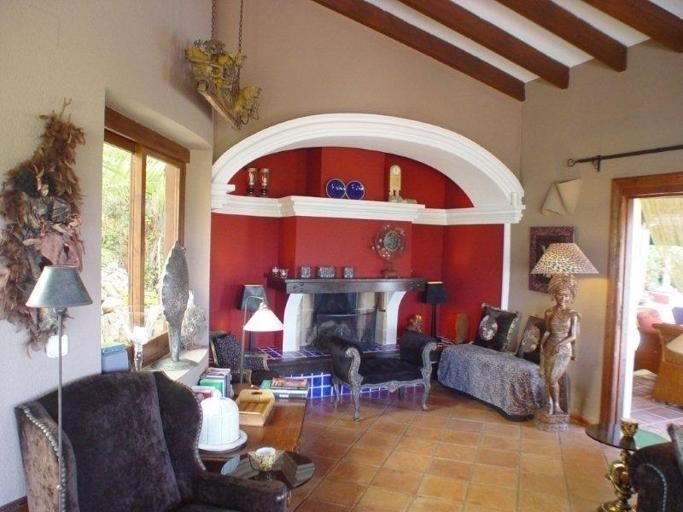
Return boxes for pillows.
[512,313,547,366]
[470,302,520,353]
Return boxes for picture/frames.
[370,223,408,262]
[526,225,574,294]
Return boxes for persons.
[538,275,582,417]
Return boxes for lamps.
[239,296,288,382]
[24,264,93,510]
[244,168,257,197]
[418,280,448,337]
[528,240,598,431]
[257,168,272,198]
[236,282,269,350]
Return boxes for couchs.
[16,369,292,512]
[629,422,682,510]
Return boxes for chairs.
[210,331,279,386]
[632,307,662,376]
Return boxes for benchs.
[440,342,544,424]
[323,330,437,423]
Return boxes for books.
[190,367,312,405]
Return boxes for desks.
[219,449,315,511]
[197,376,310,465]
[583,423,671,512]
